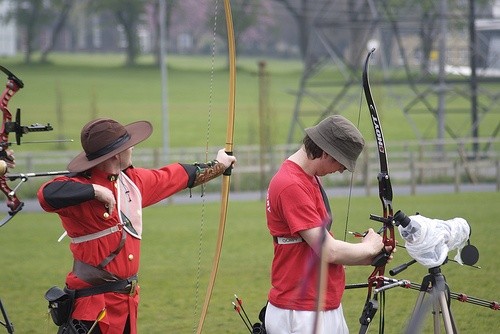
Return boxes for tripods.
[403,267,458,334]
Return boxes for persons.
[34,116,236,334]
[266,113,395,334]
[0,146,17,176]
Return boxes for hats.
[303,114,367,174]
[66,117,153,175]
